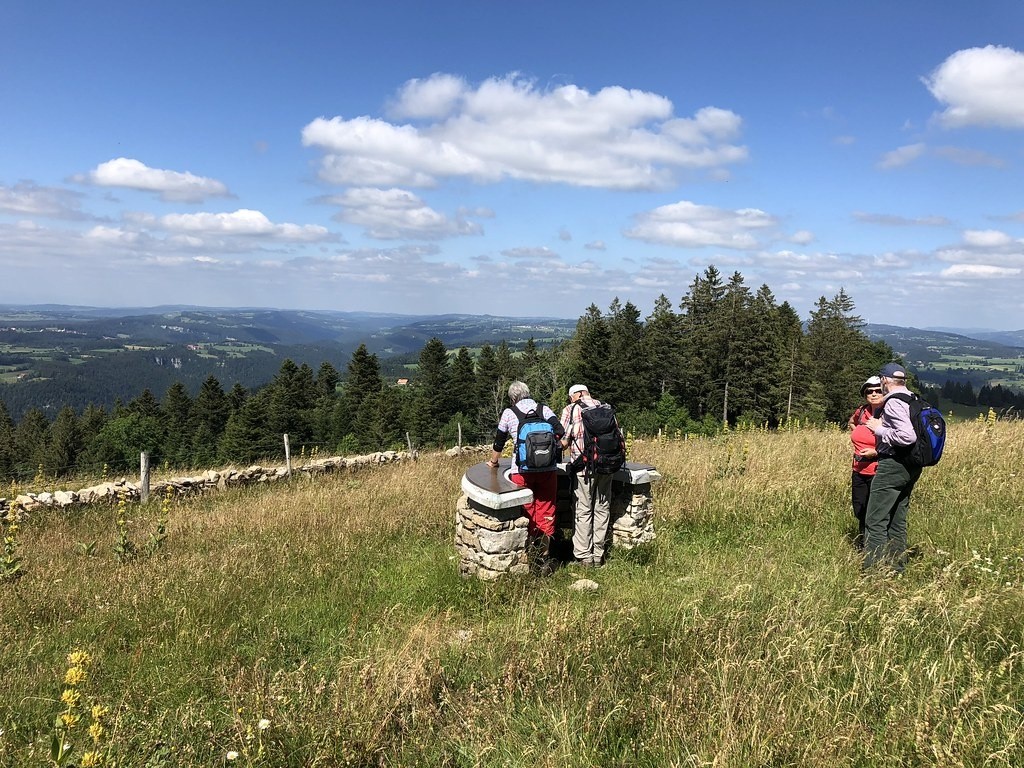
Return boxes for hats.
[860,376,881,397]
[568,384,589,396]
[878,363,907,379]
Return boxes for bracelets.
[490,460,498,464]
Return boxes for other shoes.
[578,560,592,568]
[541,554,550,562]
[594,562,601,568]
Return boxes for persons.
[487,381,565,564]
[847,362,921,572]
[557,384,619,568]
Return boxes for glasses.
[866,389,882,394]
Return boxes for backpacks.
[506,403,563,467]
[875,393,946,467]
[570,400,624,474]
